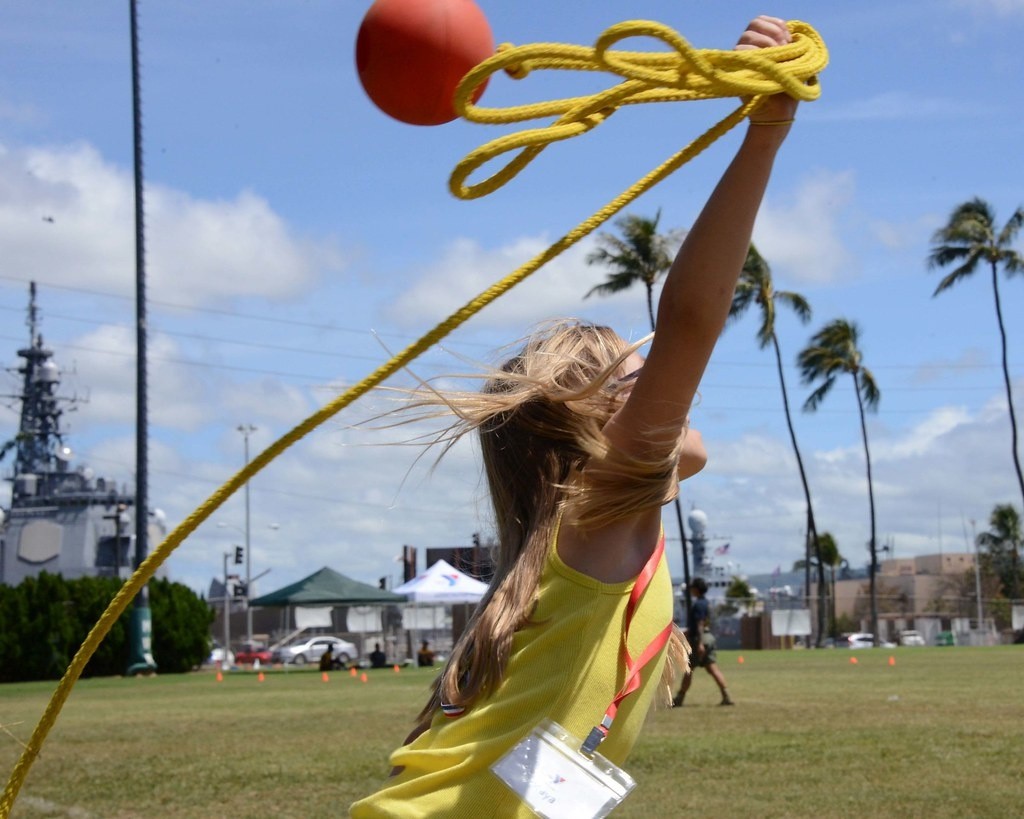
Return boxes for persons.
[350,15,800,819]
[320,645,345,671]
[418,641,436,666]
[370,644,386,668]
[671,578,734,706]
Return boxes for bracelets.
[748,118,797,126]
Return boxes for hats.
[689,578,706,594]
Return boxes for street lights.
[100,502,132,579]
[236,423,260,639]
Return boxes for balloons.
[355,1,494,126]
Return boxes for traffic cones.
[215,671,223,682]
[393,664,401,673]
[350,667,357,675]
[737,656,744,663]
[359,672,368,682]
[888,655,896,665]
[848,655,856,664]
[257,670,264,681]
[320,672,328,682]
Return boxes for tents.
[246,567,418,670]
[384,559,491,657]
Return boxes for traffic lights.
[234,545,243,565]
[234,585,247,596]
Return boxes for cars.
[898,629,926,646]
[235,642,273,665]
[272,634,359,665]
[821,631,896,648]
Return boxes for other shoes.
[670,699,682,706]
[716,701,735,707]
